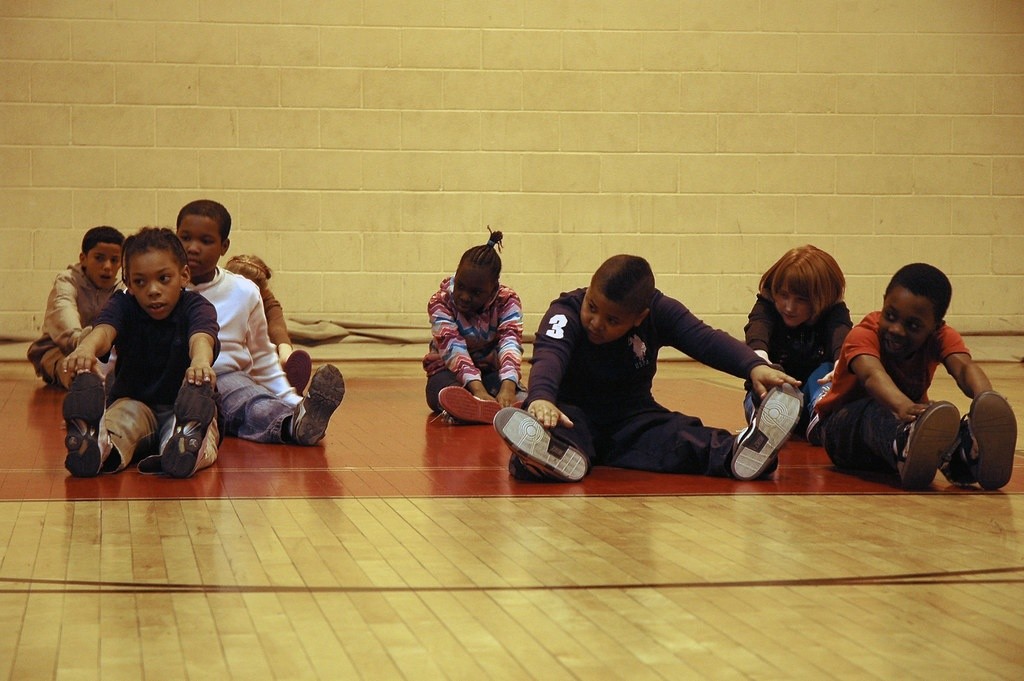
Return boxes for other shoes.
[968,390,1017,489]
[493,408,587,481]
[429,386,502,423]
[160,380,215,477]
[285,350,311,396]
[62,372,112,478]
[512,400,526,411]
[806,386,835,445]
[731,384,804,479]
[893,400,960,490]
[290,364,346,445]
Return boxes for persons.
[62,228,225,478]
[743,245,854,445]
[493,254,804,482]
[176,200,345,446]
[224,254,311,396]
[422,226,530,424]
[816,262,1017,490]
[27,226,126,389]
